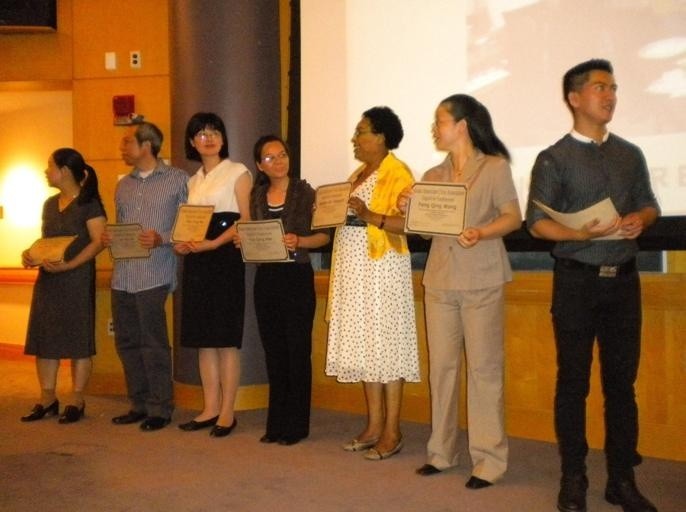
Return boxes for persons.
[396,94,522,488]
[100,120,191,432]
[171,112,255,437]
[310,106,424,460]
[525,59,660,512]
[232,134,334,445]
[21,147,109,426]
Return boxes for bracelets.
[296,235,300,249]
[377,215,386,229]
[399,214,406,219]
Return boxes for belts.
[561,256,636,278]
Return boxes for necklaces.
[449,155,475,178]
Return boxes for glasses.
[197,131,221,139]
[261,152,287,162]
